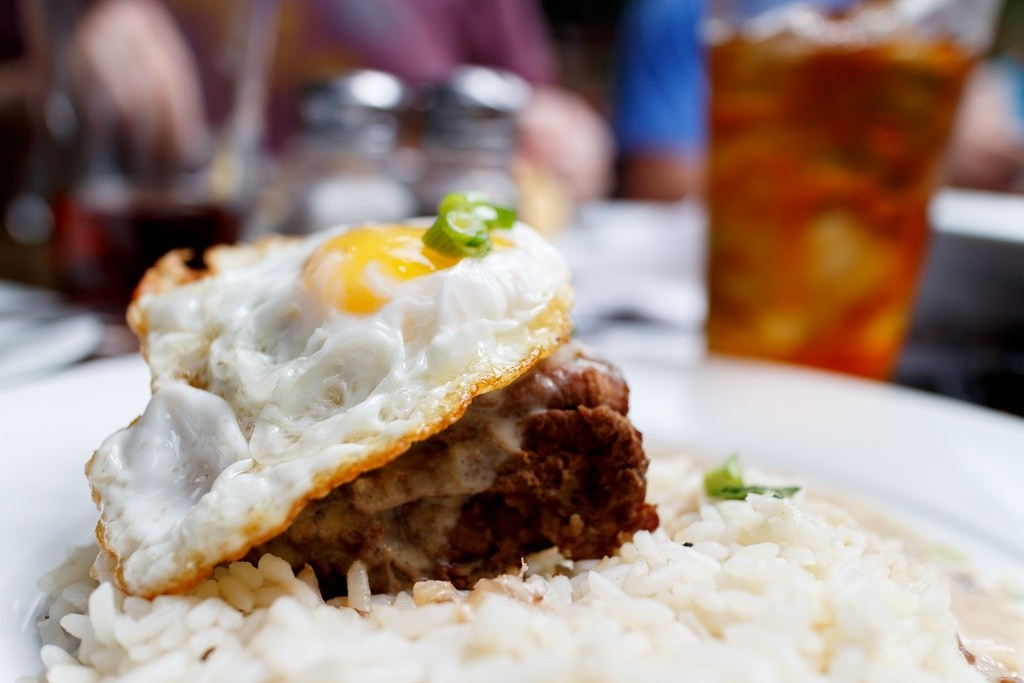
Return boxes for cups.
[18,0,282,323]
[695,0,984,382]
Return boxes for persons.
[615,0,1024,205]
[35,1,561,213]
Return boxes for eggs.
[83,200,580,598]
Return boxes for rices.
[32,459,989,683]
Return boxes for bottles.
[260,63,411,252]
[416,56,528,225]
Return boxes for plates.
[0,319,1024,681]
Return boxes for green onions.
[422,188,518,262]
[701,466,802,498]
